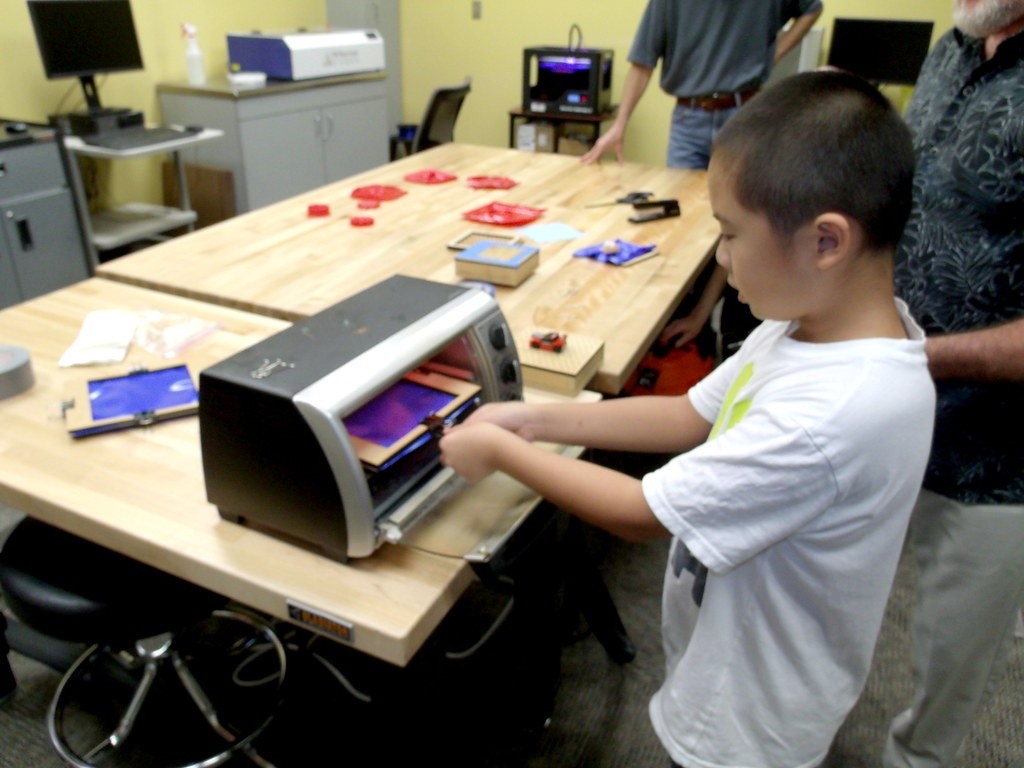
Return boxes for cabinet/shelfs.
[0,118,96,309]
[155,71,390,216]
[63,122,225,250]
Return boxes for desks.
[92,143,722,396]
[0,277,604,705]
[507,103,620,153]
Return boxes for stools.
[0,515,290,768]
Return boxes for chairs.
[410,75,472,153]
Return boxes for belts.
[678,88,758,112]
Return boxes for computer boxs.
[48,110,143,137]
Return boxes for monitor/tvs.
[28,0,143,118]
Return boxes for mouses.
[187,126,205,132]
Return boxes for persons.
[438,73,936,768]
[581,0,824,170]
[661,264,764,368]
[880,0,1024,768]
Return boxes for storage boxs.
[515,123,554,152]
[558,136,593,156]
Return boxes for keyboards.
[85,126,187,150]
